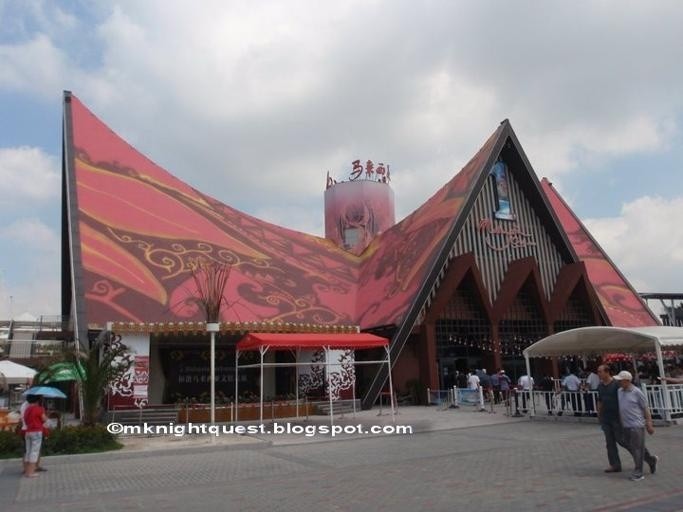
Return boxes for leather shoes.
[604,465,622,473]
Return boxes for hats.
[613,370,632,381]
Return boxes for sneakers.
[650,455,659,474]
[629,472,645,481]
[22,467,48,477]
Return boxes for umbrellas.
[20,386,68,407]
[29,360,86,427]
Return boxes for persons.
[21,396,49,478]
[595,364,626,473]
[19,395,44,471]
[557,365,681,418]
[449,364,511,407]
[538,373,555,416]
[610,370,658,482]
[516,371,535,412]
